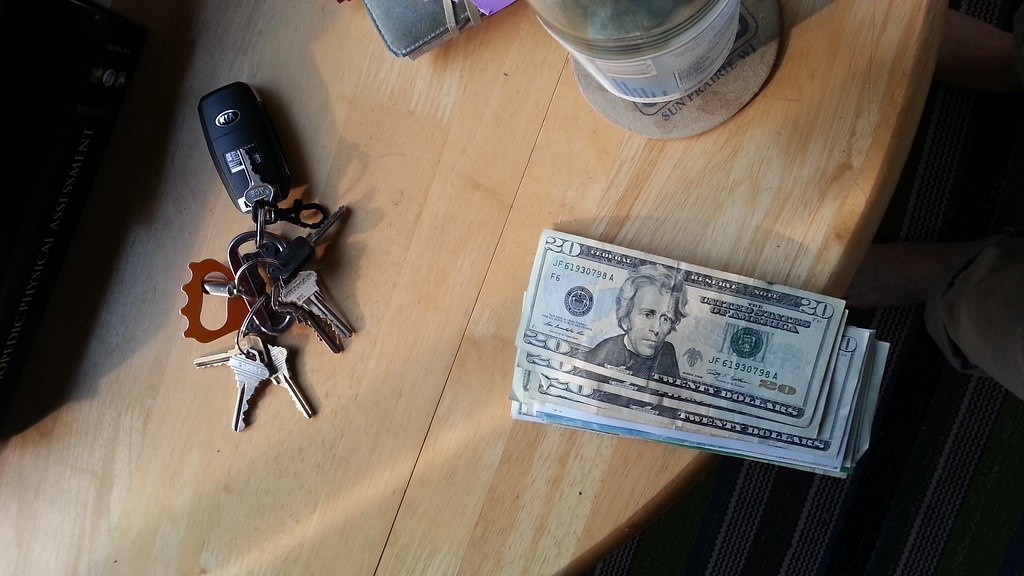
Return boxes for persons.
[845,8,1024,403]
[579,264,691,384]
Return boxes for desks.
[0,0,1017,576]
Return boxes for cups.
[526,0,742,104]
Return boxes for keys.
[194,80,355,432]
